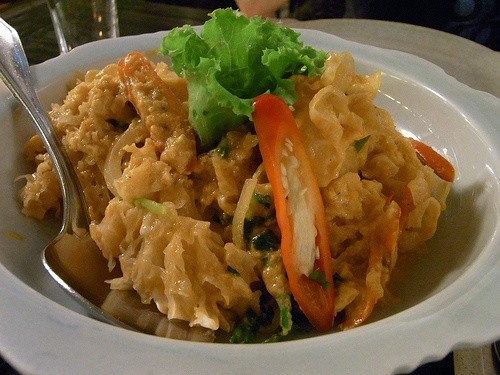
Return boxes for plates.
[1,22,499,375]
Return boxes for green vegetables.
[15,6,457,343]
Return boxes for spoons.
[0,15,139,333]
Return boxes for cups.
[45,0,122,56]
